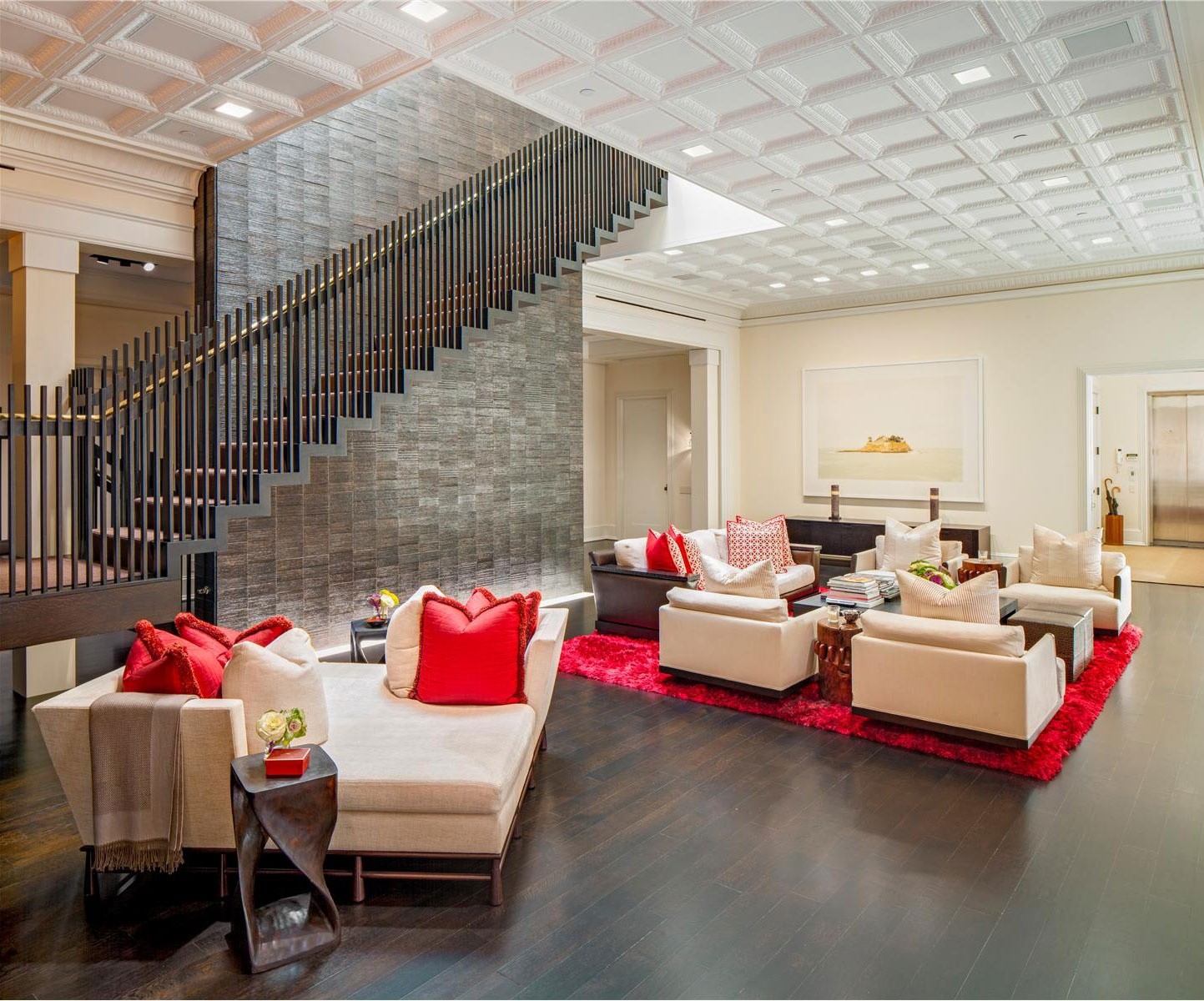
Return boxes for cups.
[978,550,988,563]
[827,605,841,628]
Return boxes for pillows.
[171,609,297,670]
[699,556,780,600]
[723,520,788,574]
[117,616,224,703]
[894,567,1012,628]
[465,586,542,646]
[382,582,448,701]
[735,513,797,568]
[218,624,333,757]
[668,524,706,591]
[881,517,944,576]
[1029,522,1105,590]
[408,590,529,707]
[644,528,688,575]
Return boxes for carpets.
[557,621,1154,783]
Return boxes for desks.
[956,555,1007,590]
[812,613,862,708]
[349,617,388,664]
[218,743,345,976]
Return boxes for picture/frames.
[795,355,994,505]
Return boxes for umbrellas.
[1105,479,1121,515]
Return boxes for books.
[821,569,900,609]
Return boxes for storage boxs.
[263,747,310,779]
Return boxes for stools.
[1006,600,1095,684]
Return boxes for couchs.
[31,601,570,913]
[851,532,969,585]
[1005,544,1134,638]
[655,585,833,703]
[586,540,827,643]
[849,608,1068,755]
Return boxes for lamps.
[829,480,843,520]
[929,485,941,523]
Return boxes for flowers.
[906,557,957,592]
[365,592,399,612]
[251,708,310,747]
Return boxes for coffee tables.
[789,588,1018,623]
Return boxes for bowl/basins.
[840,609,862,622]
